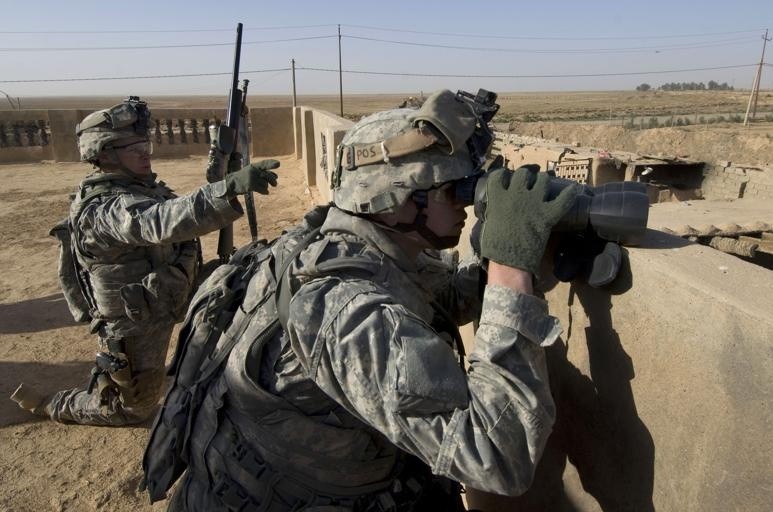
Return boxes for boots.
[9,382,51,417]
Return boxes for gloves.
[225,158,280,196]
[478,163,578,287]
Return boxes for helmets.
[78,110,153,163]
[333,108,483,216]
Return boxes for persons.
[9,96,281,427]
[168,89,579,512]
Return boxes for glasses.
[420,182,456,206]
[108,137,155,158]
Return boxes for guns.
[206,22,249,256]
